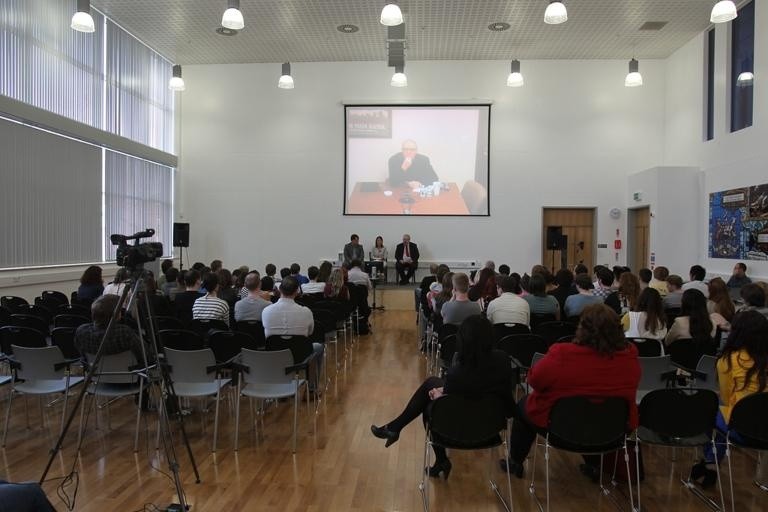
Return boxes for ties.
[405,245,409,258]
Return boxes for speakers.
[173,223,189,247]
[546,225,562,250]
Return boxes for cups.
[383,183,394,196]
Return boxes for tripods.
[39,269,200,512]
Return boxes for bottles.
[371,267,377,278]
[400,193,416,214]
[416,182,448,200]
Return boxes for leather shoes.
[303,390,322,401]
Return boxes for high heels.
[690,457,720,488]
[425,460,452,480]
[370,424,400,448]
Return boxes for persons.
[348,110,388,130]
[371,260,768,489]
[396,234,419,284]
[384,140,440,190]
[341,234,366,271]
[75,259,372,401]
[371,236,389,277]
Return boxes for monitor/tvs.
[365,261,383,279]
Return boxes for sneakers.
[499,457,525,479]
